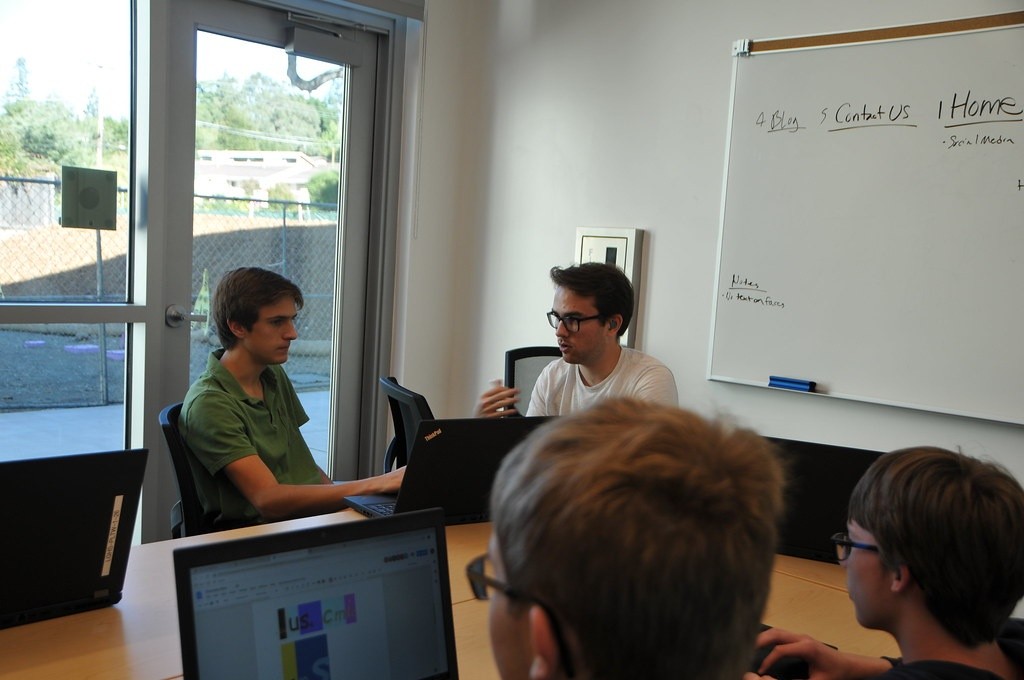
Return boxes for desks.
[0,510,901,680]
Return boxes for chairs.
[765,436,886,564]
[158,402,213,539]
[379,375,434,474]
[504,346,563,418]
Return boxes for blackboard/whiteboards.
[705,12,1024,427]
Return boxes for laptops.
[0,447,150,631]
[172,506,459,680]
[342,416,560,520]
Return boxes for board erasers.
[768,375,817,393]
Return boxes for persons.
[472,263,680,418]
[743,446,1024,679]
[465,394,784,679]
[177,266,406,530]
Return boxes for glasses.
[547,311,623,333]
[465,554,576,680]
[830,533,926,591]
